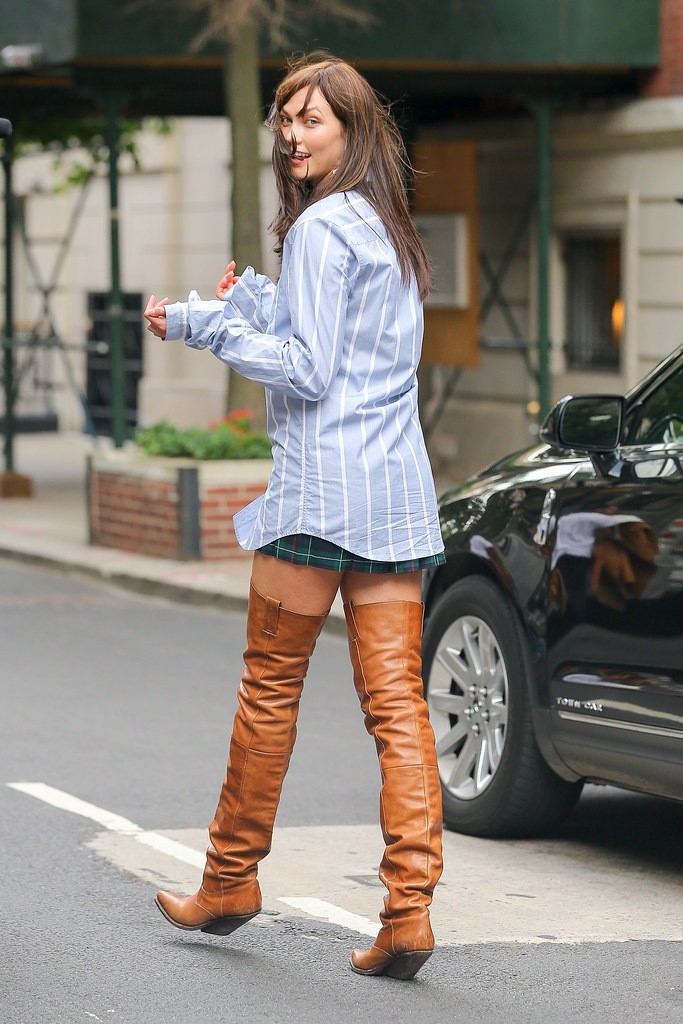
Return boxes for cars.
[421,343,682,839]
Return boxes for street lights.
[0,152,33,500]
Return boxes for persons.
[144,56,448,981]
[550,501,658,620]
[465,531,499,565]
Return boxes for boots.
[153,580,327,935]
[342,597,444,981]
